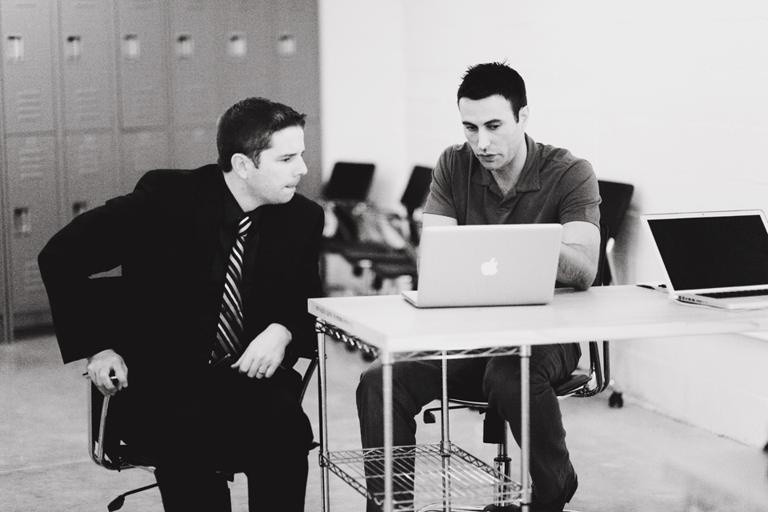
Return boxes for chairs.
[87,276,319,512]
[414,212,609,512]
[316,162,634,408]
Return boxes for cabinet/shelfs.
[0,0,321,341]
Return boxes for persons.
[35,94,327,512]
[353,59,605,511]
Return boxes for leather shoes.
[529,470,578,512]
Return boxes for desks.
[307,284,768,512]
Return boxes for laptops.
[401,223,564,308]
[640,208,768,310]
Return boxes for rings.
[95,383,99,387]
[256,369,266,376]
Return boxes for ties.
[207,216,253,370]
